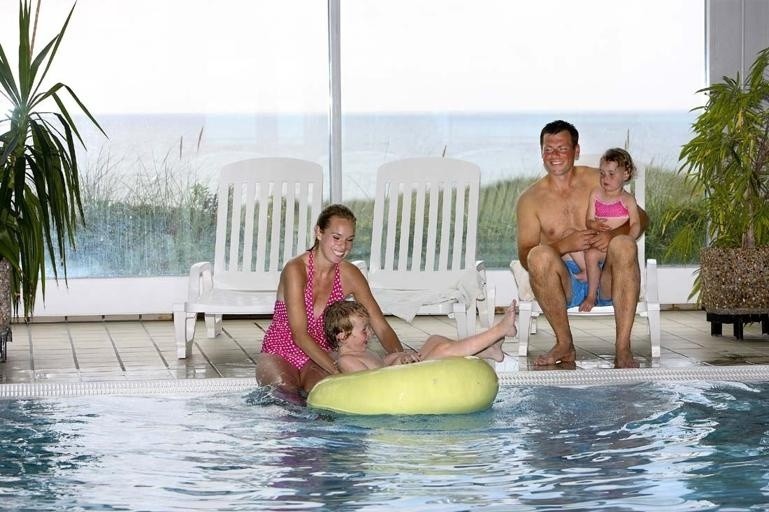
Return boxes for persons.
[517,119,649,369]
[562,147,641,313]
[253,202,404,406]
[322,298,517,374]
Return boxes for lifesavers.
[306,355,497,414]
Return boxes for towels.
[368,270,486,322]
[510,259,536,301]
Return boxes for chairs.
[518,152,661,358]
[172,157,324,359]
[351,156,496,343]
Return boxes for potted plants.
[0,0,108,334]
[661,47,769,315]
